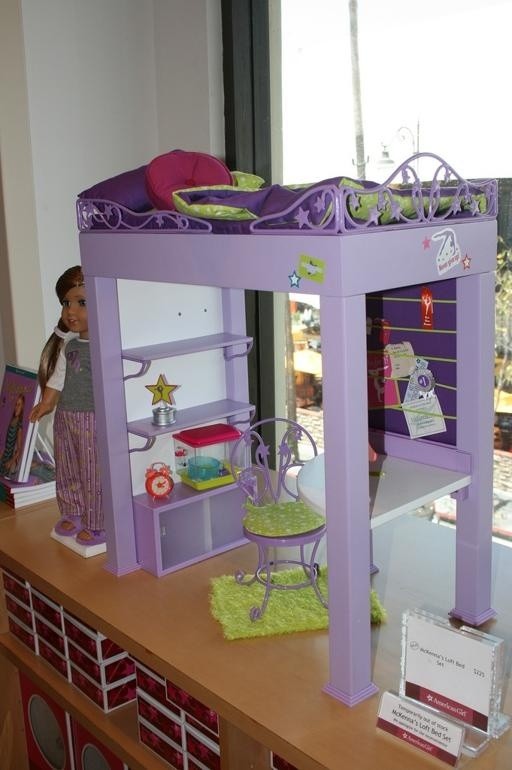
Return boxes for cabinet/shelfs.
[118,331,259,453]
[0,459,512,768]
[131,464,262,578]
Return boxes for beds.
[73,138,506,242]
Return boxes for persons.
[26,266,106,545]
[0,393,25,480]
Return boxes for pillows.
[144,150,234,208]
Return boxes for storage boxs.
[0,568,134,717]
[128,661,221,770]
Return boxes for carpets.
[207,565,391,640]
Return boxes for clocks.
[143,461,175,505]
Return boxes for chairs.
[219,412,327,623]
